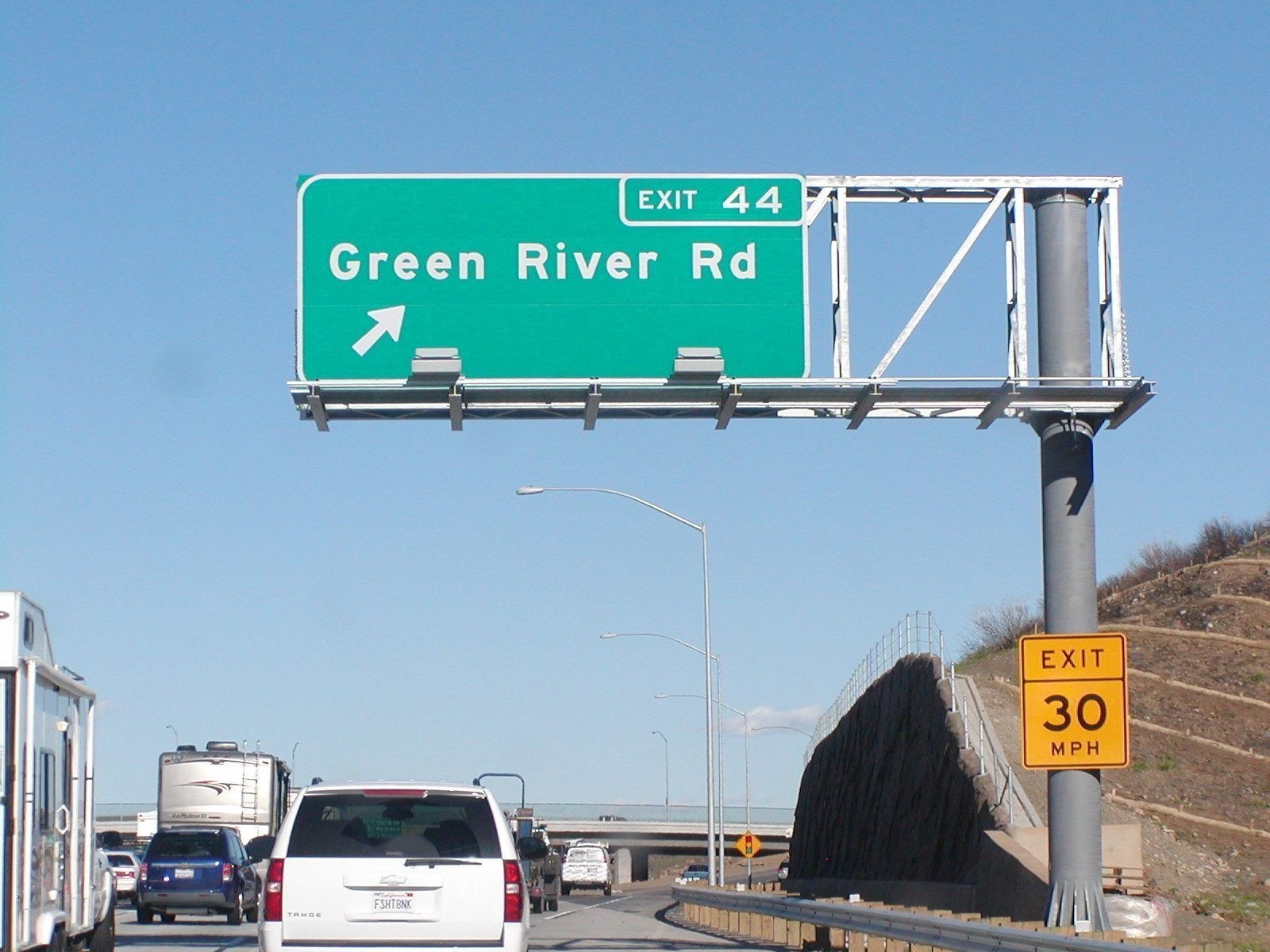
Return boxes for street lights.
[653,694,755,890]
[596,630,728,891]
[512,484,718,886]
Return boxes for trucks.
[0,590,121,952]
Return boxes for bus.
[152,738,291,891]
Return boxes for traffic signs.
[296,166,808,395]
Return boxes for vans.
[559,842,617,897]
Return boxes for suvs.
[136,825,262,927]
[242,777,550,951]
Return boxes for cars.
[98,849,143,900]
[680,864,718,883]
[776,859,790,882]
